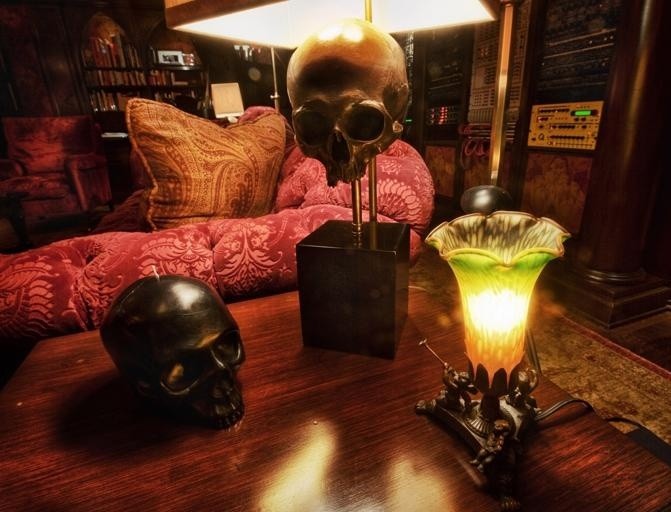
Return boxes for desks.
[0,279,671,512]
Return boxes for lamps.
[163,0,497,222]
[415,210,572,512]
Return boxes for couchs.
[0,114,436,340]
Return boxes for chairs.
[0,114,114,254]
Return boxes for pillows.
[126,95,287,233]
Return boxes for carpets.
[408,241,671,446]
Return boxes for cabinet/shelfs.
[70,13,155,129]
[517,1,625,237]
[456,1,537,213]
[419,24,471,206]
[144,13,215,125]
